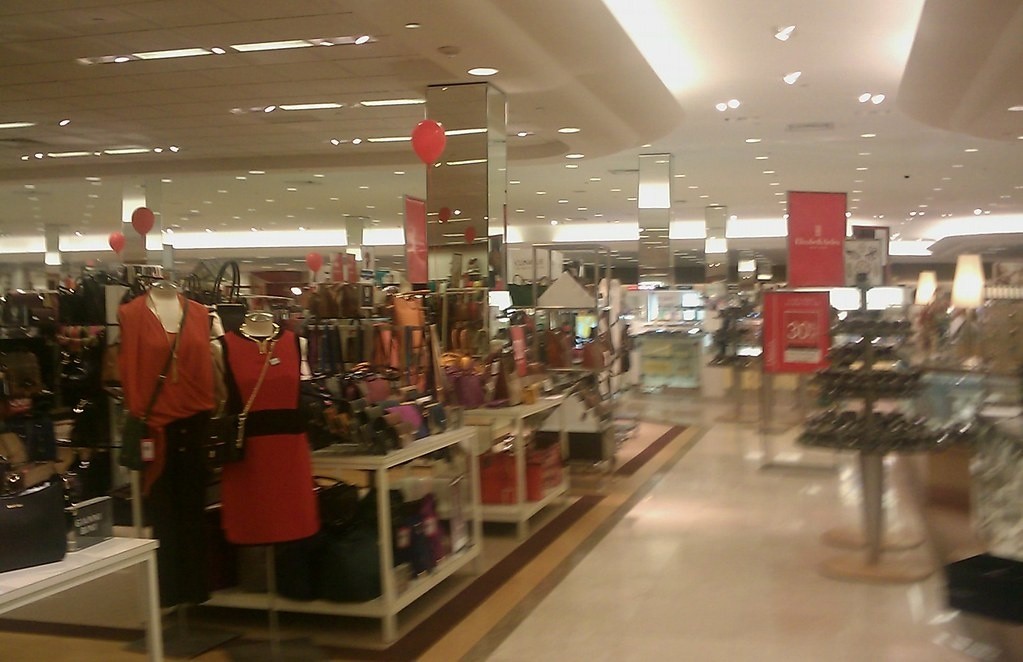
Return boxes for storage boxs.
[945,553,1023,625]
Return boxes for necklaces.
[149,293,182,383]
[240,322,279,354]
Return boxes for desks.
[0,535,164,662]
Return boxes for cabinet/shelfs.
[195,425,482,645]
[432,394,571,541]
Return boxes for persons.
[221,311,318,547]
[119,279,243,604]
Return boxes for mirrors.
[426,81,488,349]
[637,152,758,287]
[488,82,509,350]
